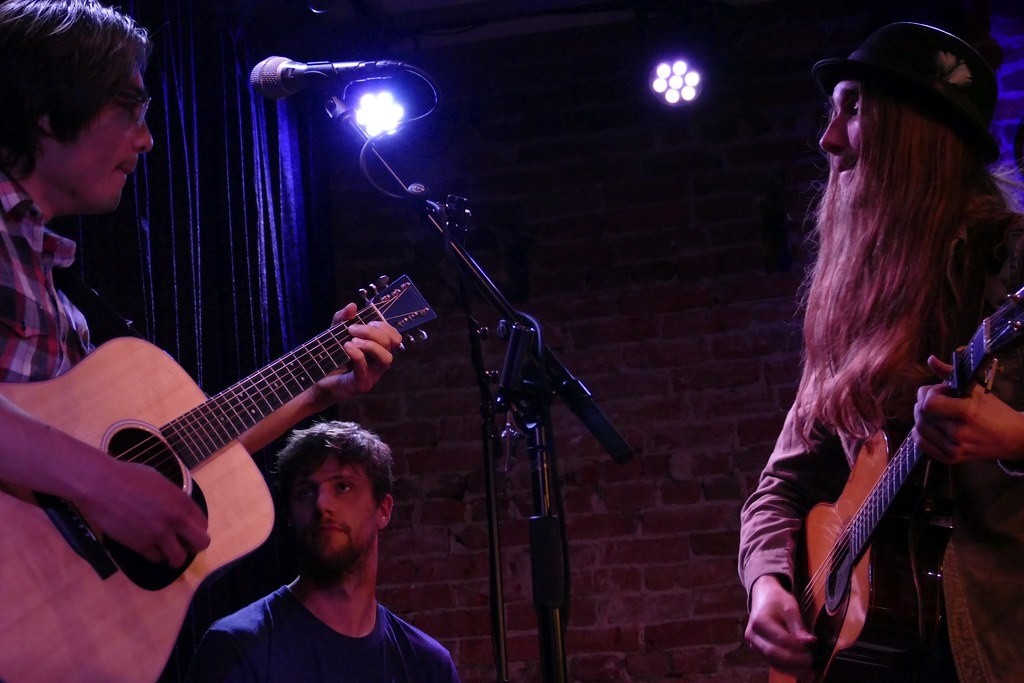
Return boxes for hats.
[810,21,1003,164]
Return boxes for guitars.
[0,273,438,682]
[767,286,1023,683]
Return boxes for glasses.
[108,88,151,128]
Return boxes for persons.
[0,2,402,586]
[189,418,461,681]
[735,20,1023,683]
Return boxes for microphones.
[249,56,405,100]
